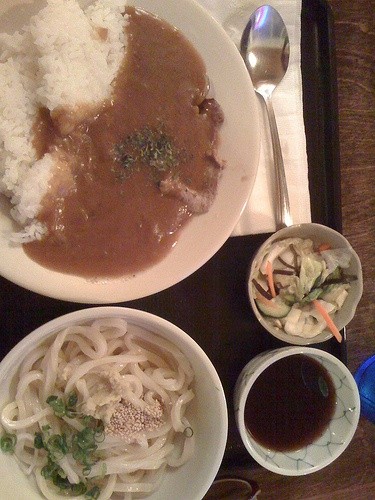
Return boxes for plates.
[0,0,262,304]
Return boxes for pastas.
[0,318,196,500]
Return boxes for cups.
[235,346,361,477]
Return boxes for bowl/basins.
[0,307,228,500]
[248,223,363,346]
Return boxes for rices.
[0,0,129,245]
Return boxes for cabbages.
[253,238,352,343]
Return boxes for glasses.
[202,476,262,500]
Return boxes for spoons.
[240,5,294,232]
